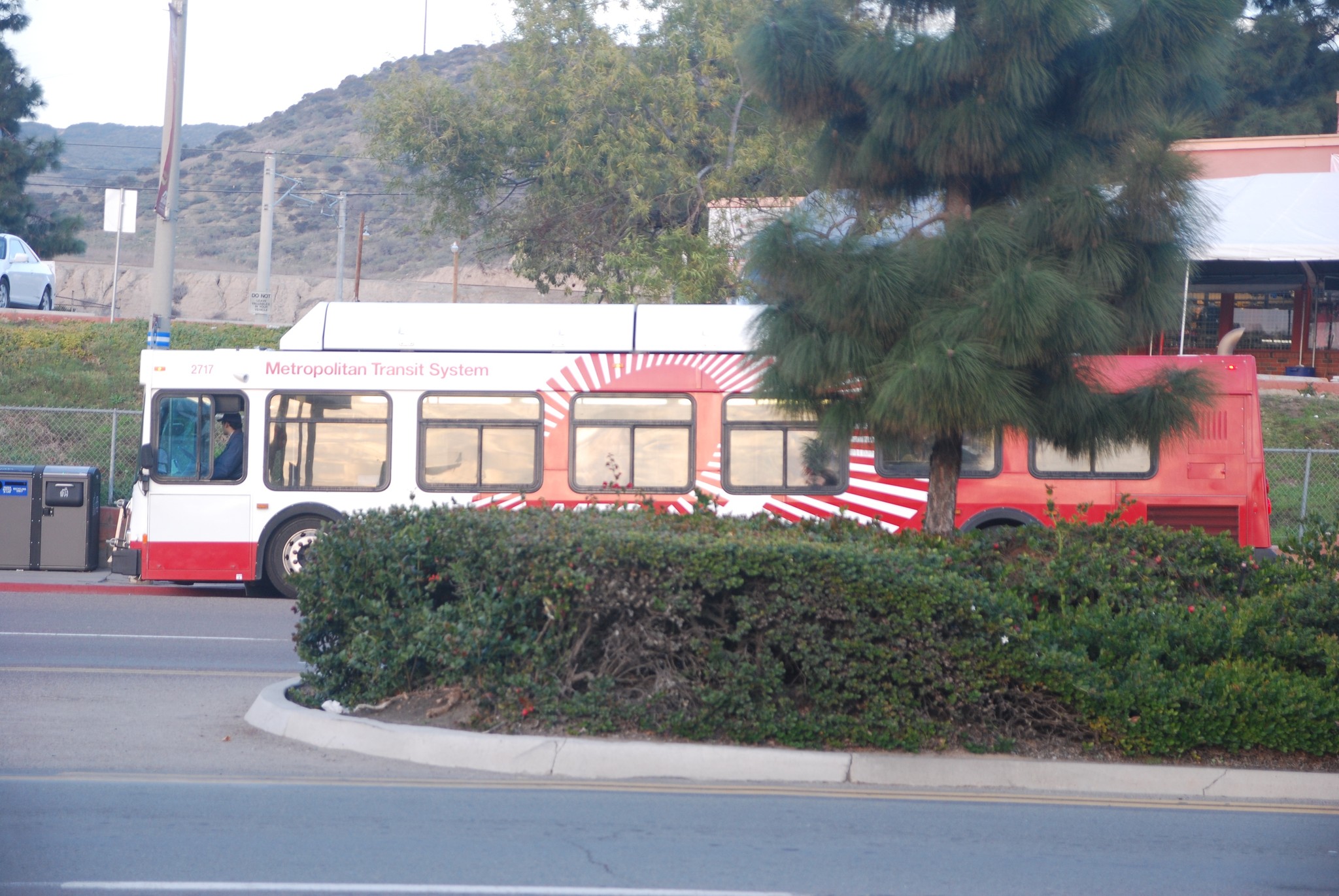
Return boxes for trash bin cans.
[0,463,101,571]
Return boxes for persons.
[202,411,244,480]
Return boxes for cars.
[0,232,55,312]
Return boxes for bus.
[139,299,1272,600]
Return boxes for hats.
[217,412,241,424]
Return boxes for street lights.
[352,211,369,302]
[450,238,459,304]
